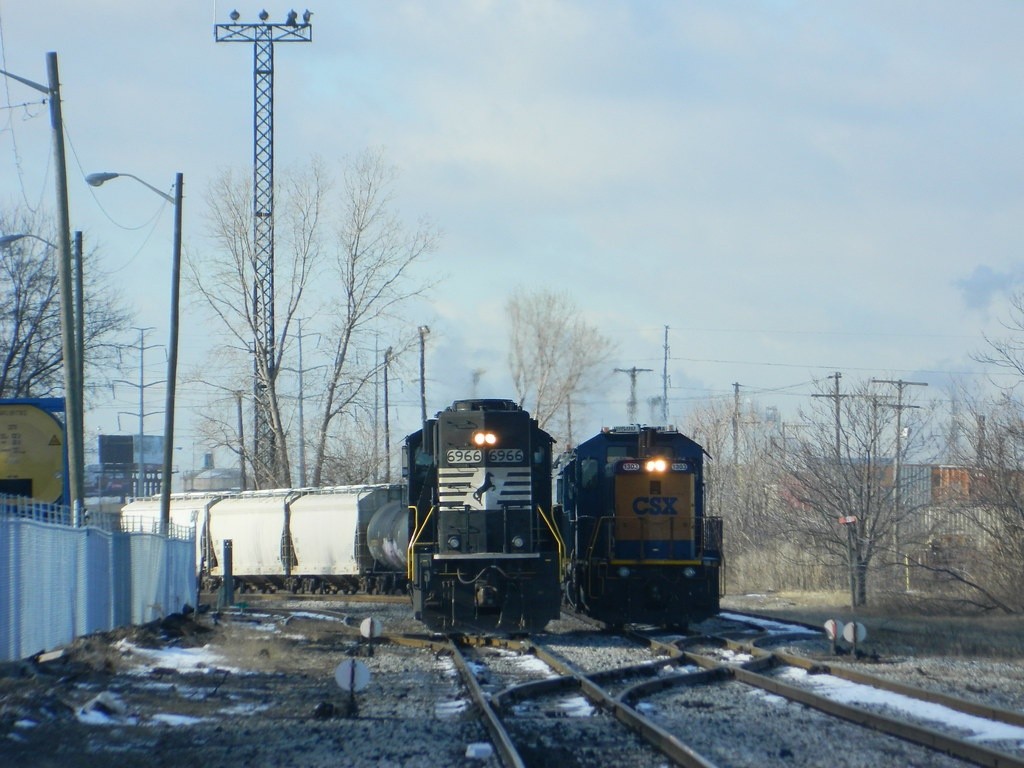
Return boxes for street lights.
[83,171,185,533]
[893,426,912,585]
[0,231,86,525]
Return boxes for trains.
[551,423,724,634]
[126,398,568,637]
